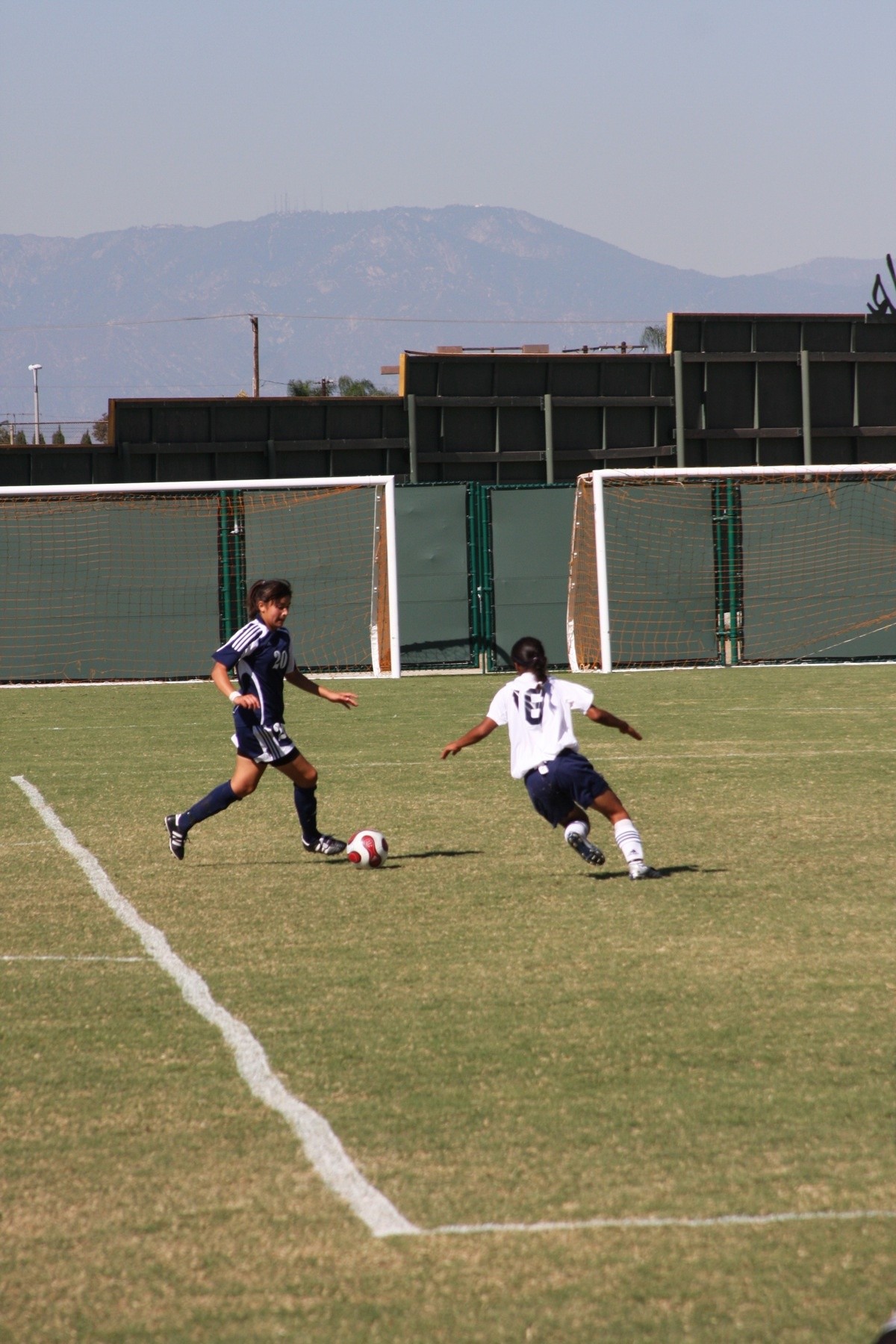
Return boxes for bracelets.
[229,691,240,703]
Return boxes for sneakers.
[166,814,188,860]
[301,834,346,856]
[567,832,605,866]
[629,867,661,881]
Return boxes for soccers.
[348,831,387,869]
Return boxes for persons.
[162,578,359,861]
[440,637,663,881]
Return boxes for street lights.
[27,363,42,446]
